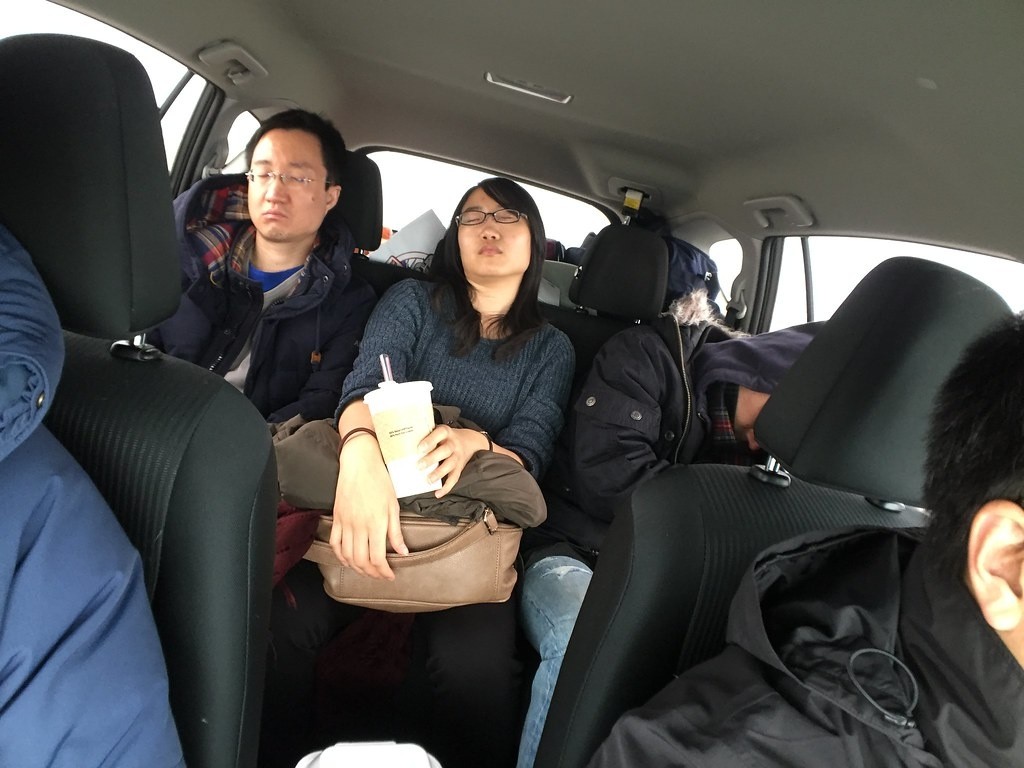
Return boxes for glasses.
[246,171,332,187]
[454,208,527,227]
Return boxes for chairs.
[336,153,383,332]
[0,34,276,768]
[543,225,673,404]
[530,255,1018,768]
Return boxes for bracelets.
[482,431,495,452]
[336,427,377,458]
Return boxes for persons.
[587,308,1023,766]
[513,314,831,768]
[146,104,392,432]
[1,212,188,767]
[272,183,582,767]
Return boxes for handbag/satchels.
[299,509,523,614]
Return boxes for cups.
[364,381,442,499]
